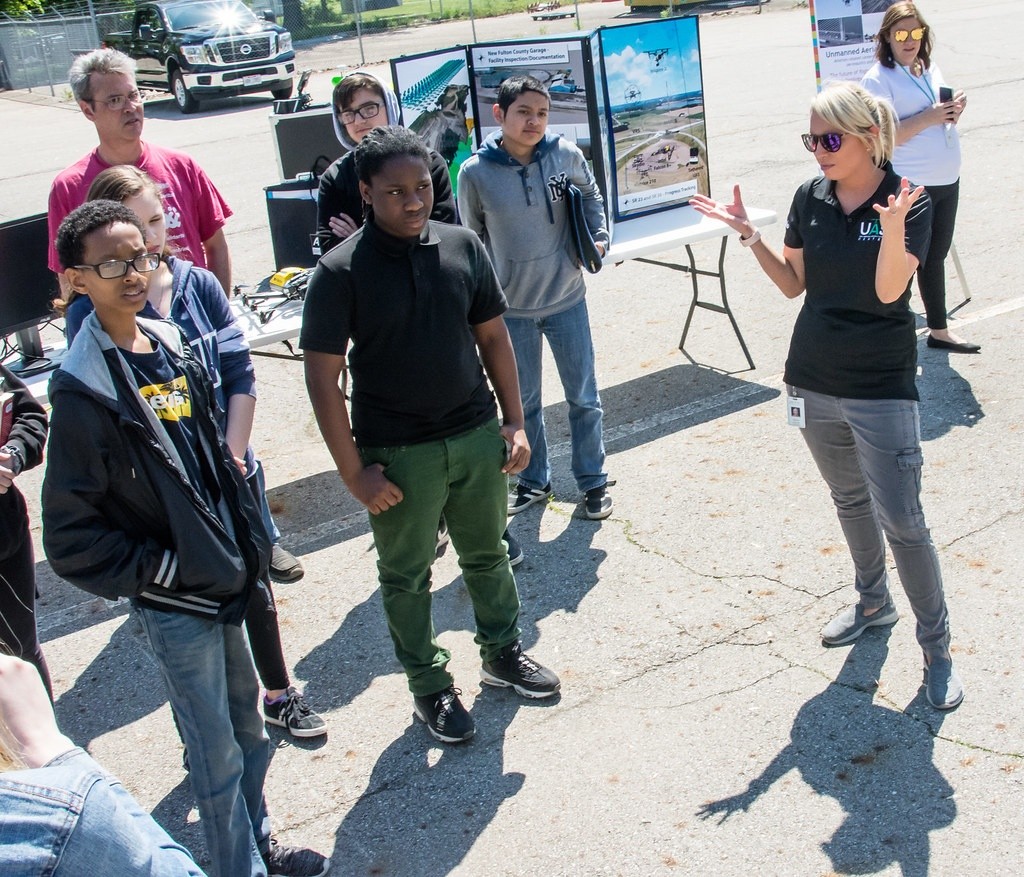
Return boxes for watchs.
[738,228,761,247]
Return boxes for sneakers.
[923,647,965,709]
[502,531,524,566]
[413,684,476,743]
[480,640,562,699]
[585,481,615,519]
[263,687,327,736]
[433,518,449,557]
[507,482,552,514]
[821,598,898,644]
[262,839,330,877]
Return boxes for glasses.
[802,133,843,152]
[336,103,379,125]
[895,28,928,42]
[89,90,148,111]
[75,253,160,279]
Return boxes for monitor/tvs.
[0,212,71,380]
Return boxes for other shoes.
[270,542,304,584]
[927,333,981,353]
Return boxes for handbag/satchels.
[564,181,603,273]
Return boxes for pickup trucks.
[99,0,296,114]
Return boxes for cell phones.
[940,87,954,121]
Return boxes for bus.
[624,0,713,12]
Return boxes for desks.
[0,288,352,407]
[597,201,778,372]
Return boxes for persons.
[48,50,305,581]
[317,71,524,569]
[687,80,964,710]
[859,1,981,354]
[42,199,330,876]
[63,164,327,737]
[0,362,205,874]
[298,125,562,740]
[458,77,614,520]
